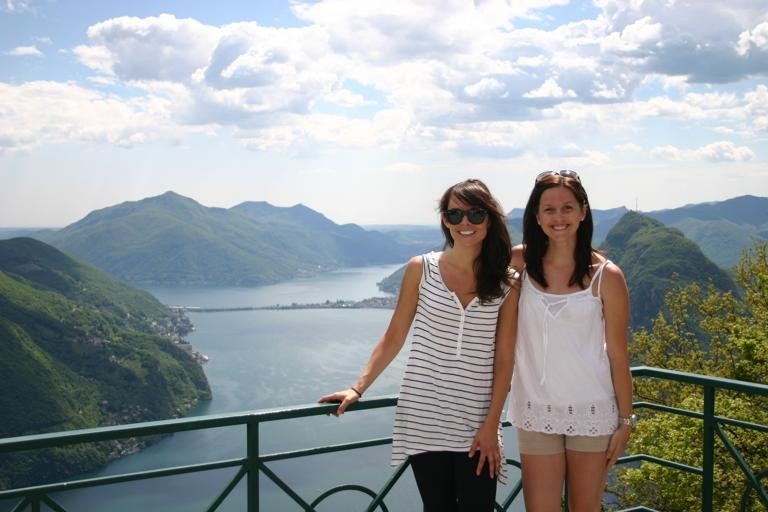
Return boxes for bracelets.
[349,387,363,398]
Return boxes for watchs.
[617,413,638,429]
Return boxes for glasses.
[441,206,490,226]
[534,170,582,186]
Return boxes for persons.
[507,170,637,511]
[316,180,522,511]
